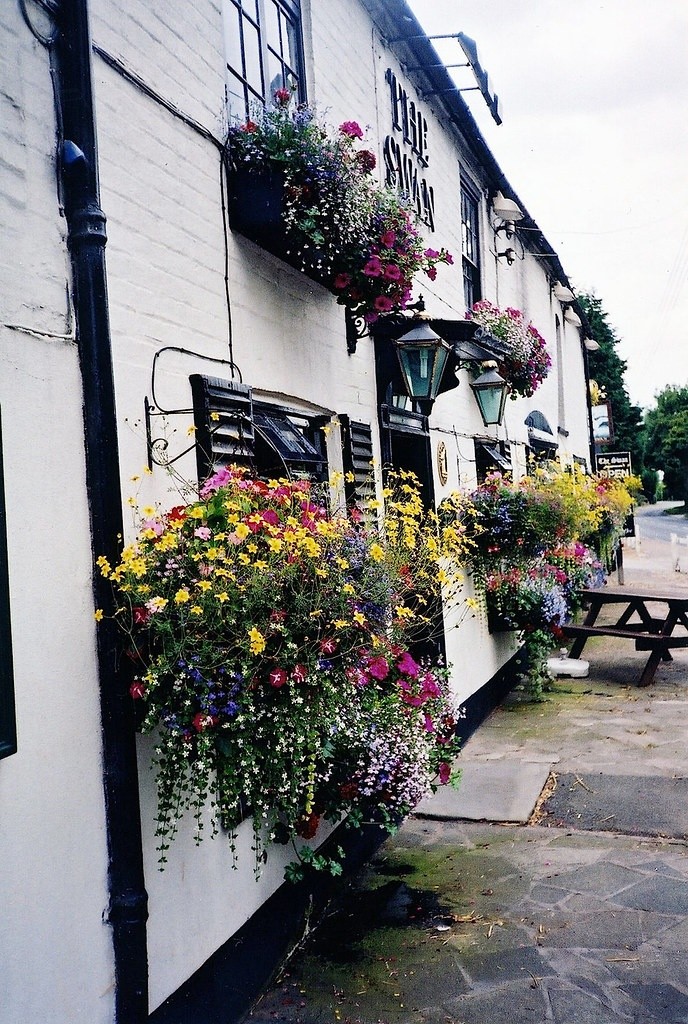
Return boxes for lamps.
[441,357,509,425]
[345,294,451,426]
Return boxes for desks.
[568,585,688,688]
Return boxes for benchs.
[562,623,670,642]
[640,616,684,626]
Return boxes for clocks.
[548,281,576,305]
[486,189,524,221]
[563,305,583,328]
[579,332,600,350]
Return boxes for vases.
[224,158,336,290]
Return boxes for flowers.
[464,301,552,401]
[230,90,454,324]
